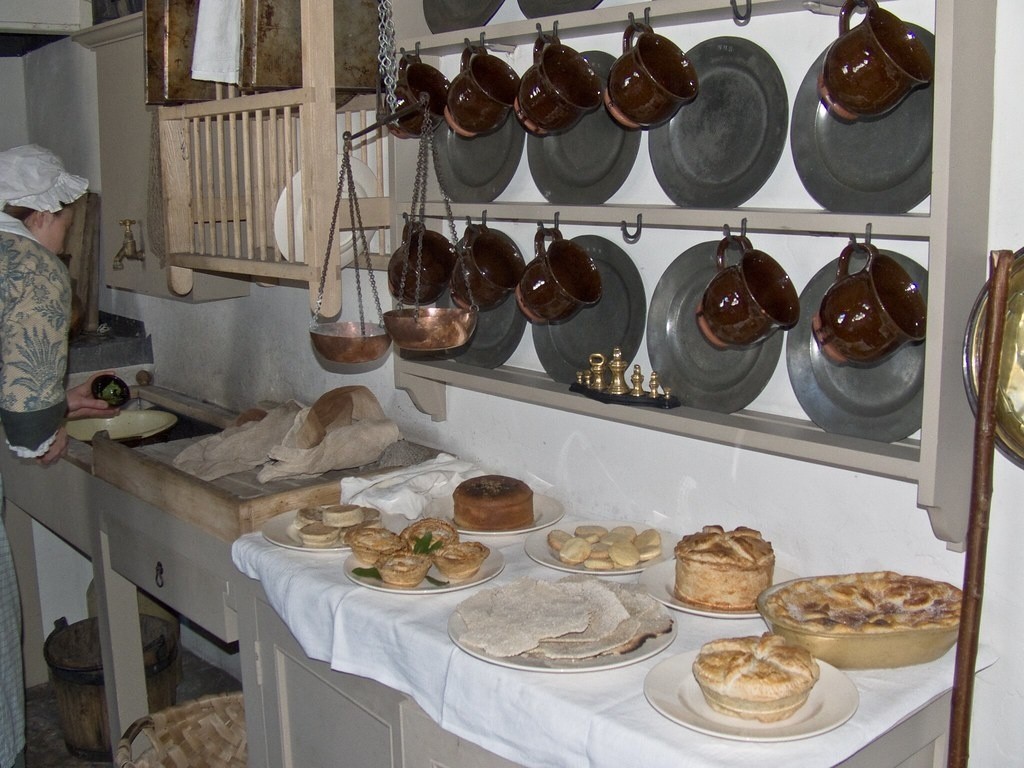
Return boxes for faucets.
[112,218,146,271]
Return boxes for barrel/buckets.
[42,613,179,762]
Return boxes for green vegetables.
[352,529,451,586]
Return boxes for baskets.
[115,689,249,768]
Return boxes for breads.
[548,524,660,569]
[452,475,533,531]
[295,503,383,548]
[767,570,963,664]
[692,630,822,723]
[673,524,777,613]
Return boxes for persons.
[0,144,122,768]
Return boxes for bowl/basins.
[91,374,130,407]
[64,410,179,445]
[755,574,960,672]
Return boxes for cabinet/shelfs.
[258,601,954,768]
[390,0,995,552]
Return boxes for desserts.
[344,517,489,579]
[373,550,432,586]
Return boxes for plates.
[422,1,506,34]
[343,538,507,596]
[431,107,526,203]
[531,235,648,385]
[789,22,934,214]
[272,154,383,271]
[452,491,565,538]
[446,578,679,673]
[638,560,803,620]
[516,0,605,20]
[648,35,789,209]
[524,519,684,577]
[643,647,861,745]
[646,241,784,416]
[786,248,929,443]
[434,286,528,370]
[261,504,352,553]
[525,50,642,206]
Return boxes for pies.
[458,574,673,659]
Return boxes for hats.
[0,145,90,214]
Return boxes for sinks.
[63,388,232,449]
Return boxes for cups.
[388,221,456,306]
[514,227,604,327]
[513,34,605,137]
[449,223,526,314]
[696,234,801,351]
[813,242,928,370]
[443,45,522,140]
[816,0,936,123]
[602,21,700,130]
[384,54,451,142]
